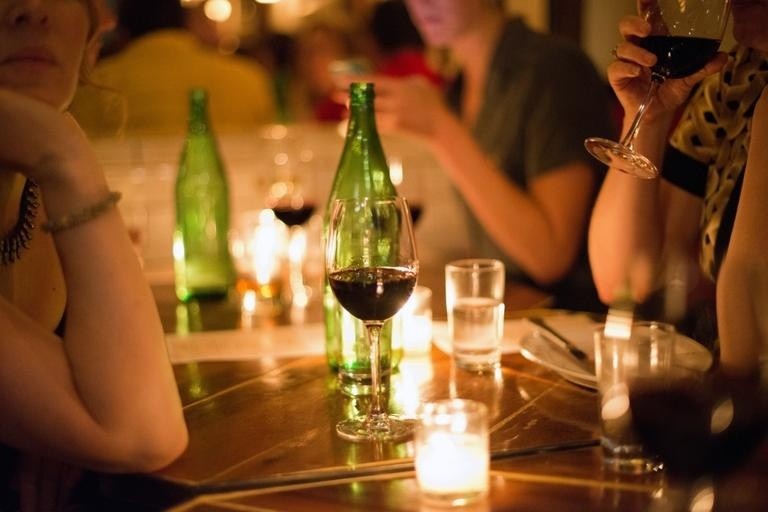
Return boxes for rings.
[608,46,620,58]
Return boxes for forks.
[523,309,596,371]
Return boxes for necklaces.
[0,177,40,267]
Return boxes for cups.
[414,398,489,512]
[444,259,506,366]
[122,201,149,248]
[594,321,679,475]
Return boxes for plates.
[515,322,715,385]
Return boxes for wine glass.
[266,150,321,305]
[583,0,729,182]
[329,198,420,442]
[369,142,427,239]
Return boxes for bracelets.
[42,191,123,235]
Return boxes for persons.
[0,0,192,474]
[586,0,768,381]
[327,0,607,313]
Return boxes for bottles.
[321,81,403,374]
[172,84,237,303]
